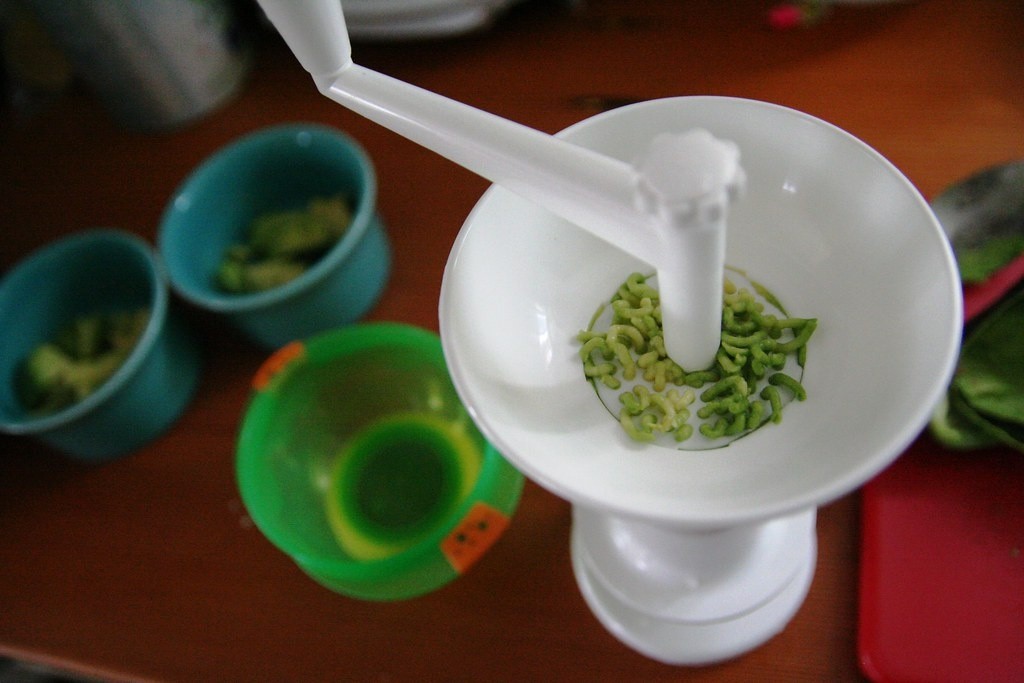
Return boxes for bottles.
[23,0,255,136]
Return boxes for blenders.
[227,1,961,665]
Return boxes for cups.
[0,223,196,462]
[158,123,391,355]
[232,320,523,602]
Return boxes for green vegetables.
[930,167,1024,451]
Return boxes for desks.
[0,0,1024,683]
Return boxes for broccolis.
[21,307,149,402]
[215,202,345,290]
